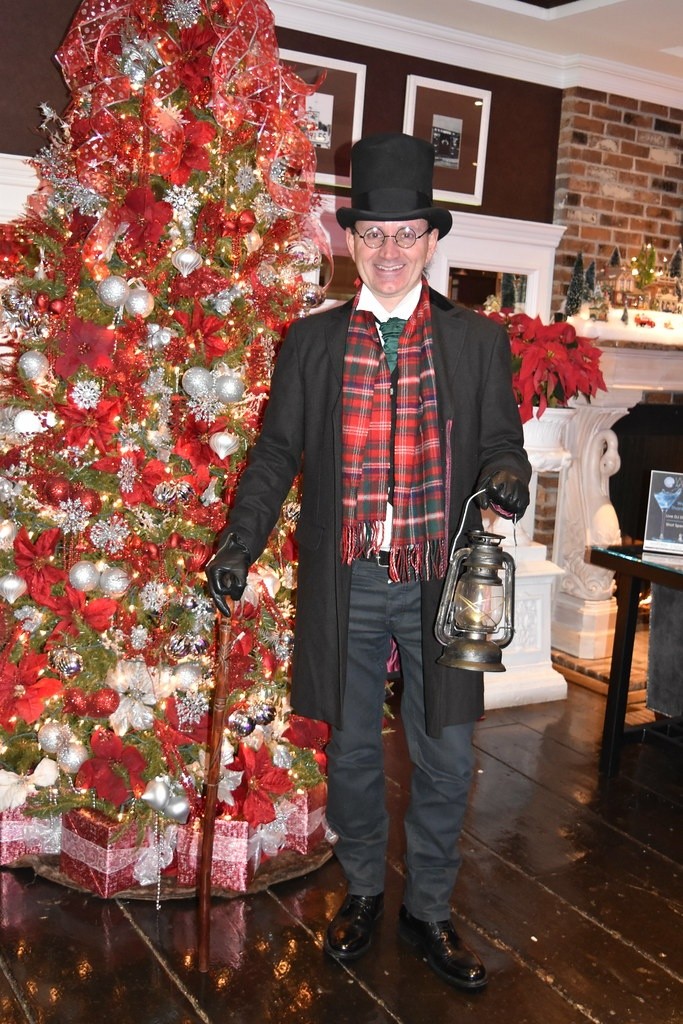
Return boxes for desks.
[589,545,683,780]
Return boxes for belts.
[356,547,415,567]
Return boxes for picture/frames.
[403,75,493,207]
[277,47,367,188]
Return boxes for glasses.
[354,224,431,249]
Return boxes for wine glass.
[652,487,681,543]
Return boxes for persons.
[205,133,532,988]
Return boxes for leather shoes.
[326,892,385,959]
[396,904,488,993]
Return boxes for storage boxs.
[1,780,328,895]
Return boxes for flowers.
[477,302,609,423]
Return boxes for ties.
[375,316,408,376]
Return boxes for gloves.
[205,533,251,618]
[477,471,530,514]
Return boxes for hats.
[336,133,452,241]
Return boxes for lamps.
[435,489,519,672]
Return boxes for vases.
[483,405,576,564]
